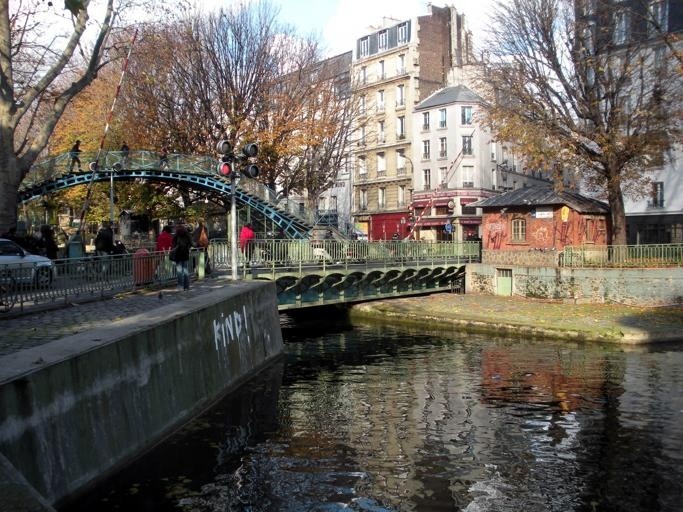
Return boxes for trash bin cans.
[133,248,154,287]
[68,240,83,274]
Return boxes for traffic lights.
[243,143,258,179]
[215,141,231,176]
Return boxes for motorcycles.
[91,240,130,274]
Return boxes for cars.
[0,239,58,292]
[207,243,401,268]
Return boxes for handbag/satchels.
[198,226,208,246]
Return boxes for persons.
[192,216,213,278]
[168,226,191,293]
[119,140,131,170]
[239,221,255,274]
[158,143,172,172]
[153,225,172,262]
[68,139,84,175]
[94,220,115,280]
[43,229,60,278]
